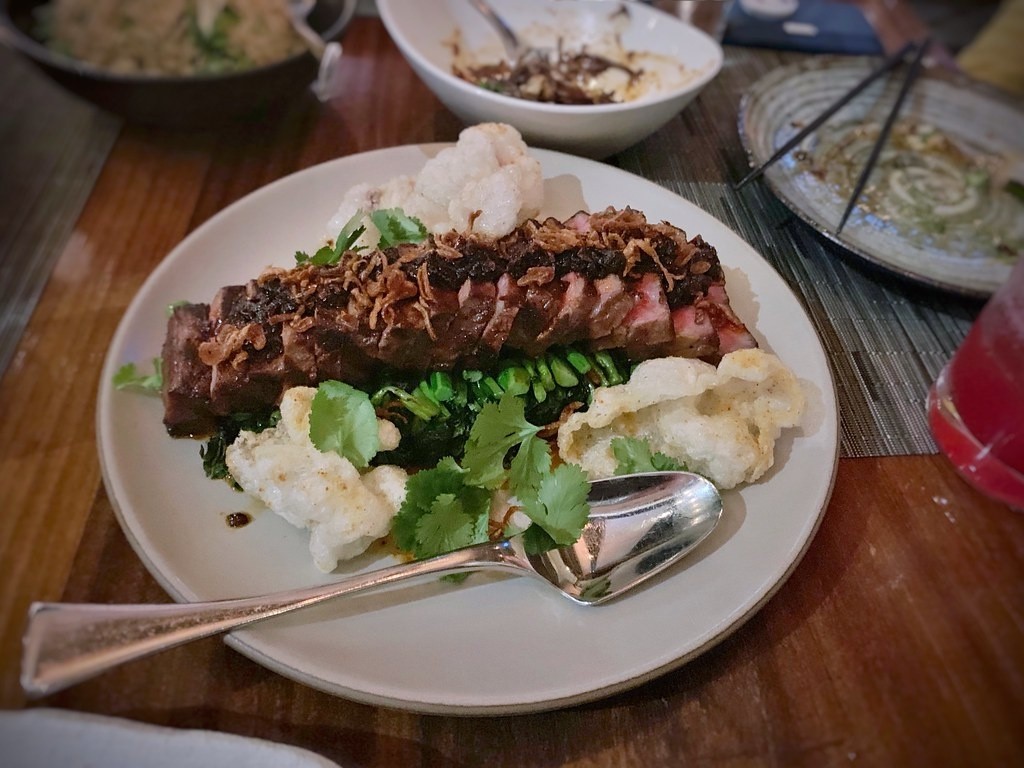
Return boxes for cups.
[924,245,1024,524]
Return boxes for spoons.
[19,468,726,701]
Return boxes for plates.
[96,141,841,718]
[737,52,1024,298]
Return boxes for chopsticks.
[732,33,934,235]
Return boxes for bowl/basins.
[374,1,723,162]
[0,1,358,120]
